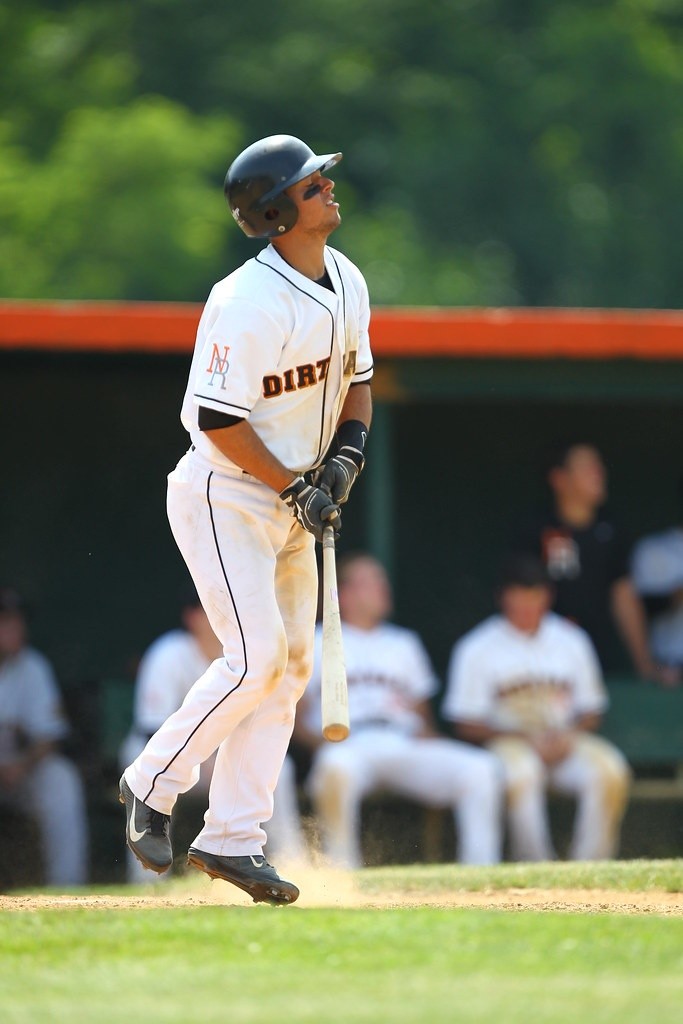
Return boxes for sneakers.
[119,773,173,874]
[188,847,300,908]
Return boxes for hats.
[496,557,553,587]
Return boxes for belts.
[191,445,250,476]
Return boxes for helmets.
[225,134,343,239]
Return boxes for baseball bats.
[311,465,351,743]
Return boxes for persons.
[117,134,374,906]
[0,440,683,882]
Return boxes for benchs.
[432,778,683,863]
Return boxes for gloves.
[281,476,343,541]
[315,446,365,502]
